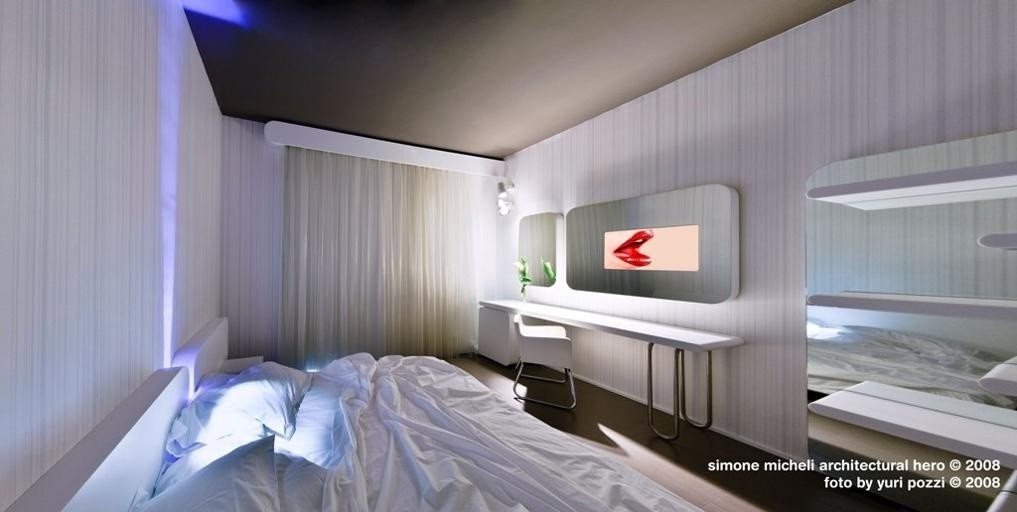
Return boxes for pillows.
[126,361,312,511]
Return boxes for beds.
[0,355,704,512]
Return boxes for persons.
[604,229,653,270]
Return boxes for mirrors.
[516,210,557,288]
[804,130,1017,511]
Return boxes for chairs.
[511,313,577,410]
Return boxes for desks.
[476,298,743,441]
